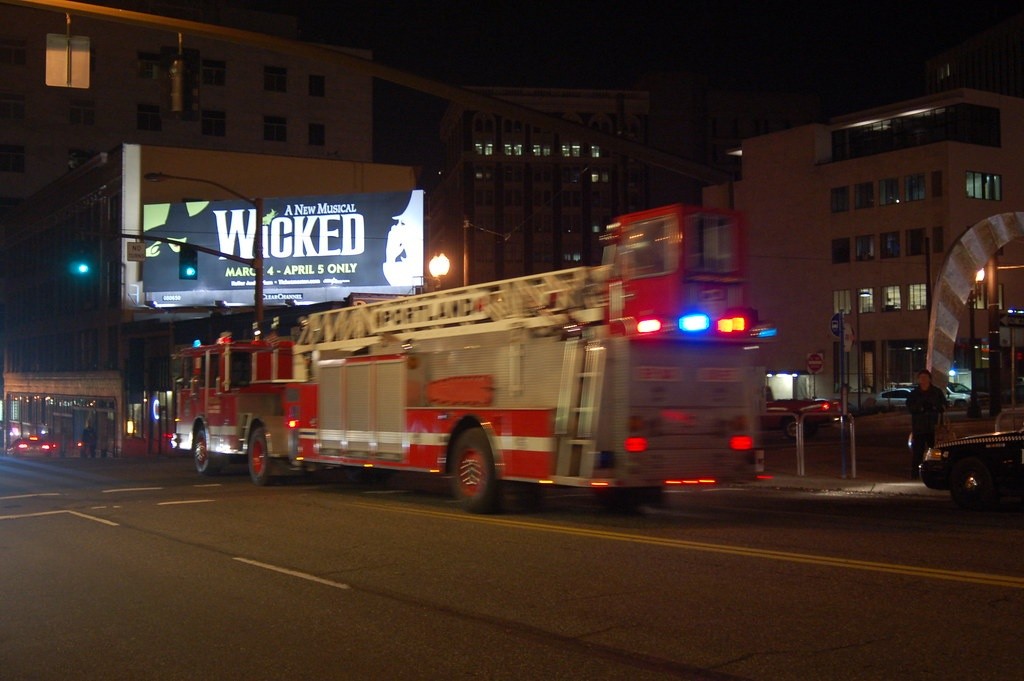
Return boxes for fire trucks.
[171,205,779,517]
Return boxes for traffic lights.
[178,245,199,281]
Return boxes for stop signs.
[806,353,824,373]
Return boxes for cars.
[876,387,912,409]
[919,429,1024,514]
[946,382,989,409]
[9,437,52,459]
[761,399,840,442]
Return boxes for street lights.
[428,253,451,289]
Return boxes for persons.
[82,429,98,459]
[906,369,948,480]
